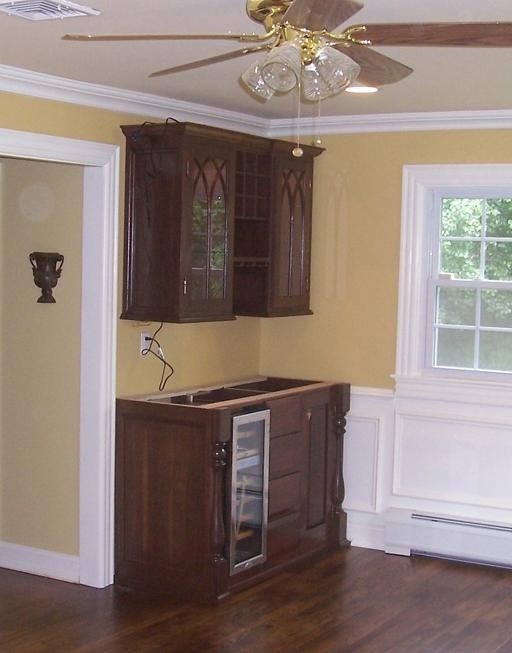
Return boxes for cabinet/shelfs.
[114,399,271,603]
[233,135,326,317]
[264,382,351,580]
[120,122,248,323]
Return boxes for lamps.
[241,37,361,158]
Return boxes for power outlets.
[140,333,150,357]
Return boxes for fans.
[61,0,512,86]
